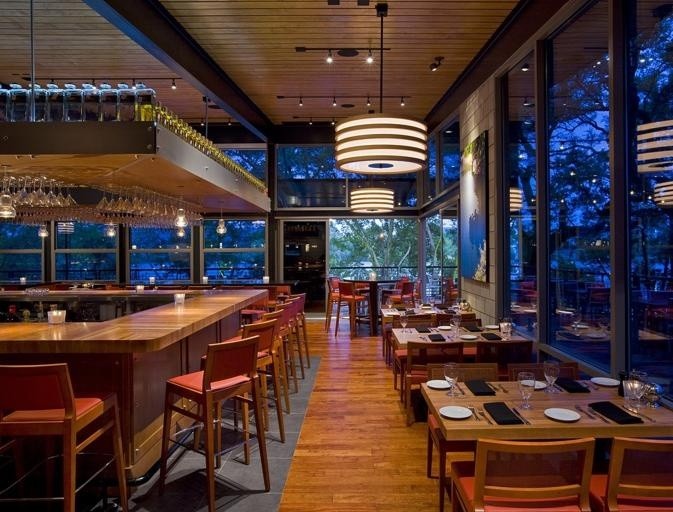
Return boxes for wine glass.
[443,361,649,416]
[0,174,204,229]
[386,297,513,342]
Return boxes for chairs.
[267,285,291,308]
[1,361,126,510]
[0,276,269,291]
[159,292,312,495]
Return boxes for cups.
[136,285,144,292]
[20,277,26,282]
[203,276,209,282]
[174,293,186,305]
[149,277,155,283]
[48,310,66,324]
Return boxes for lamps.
[365,48,375,64]
[324,50,335,63]
[348,174,395,214]
[277,96,412,127]
[637,119,673,173]
[333,3,429,173]
[654,182,672,206]
[509,188,523,212]
[0,168,227,237]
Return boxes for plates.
[426,376,620,421]
[386,306,500,340]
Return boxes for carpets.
[92,355,321,512]
